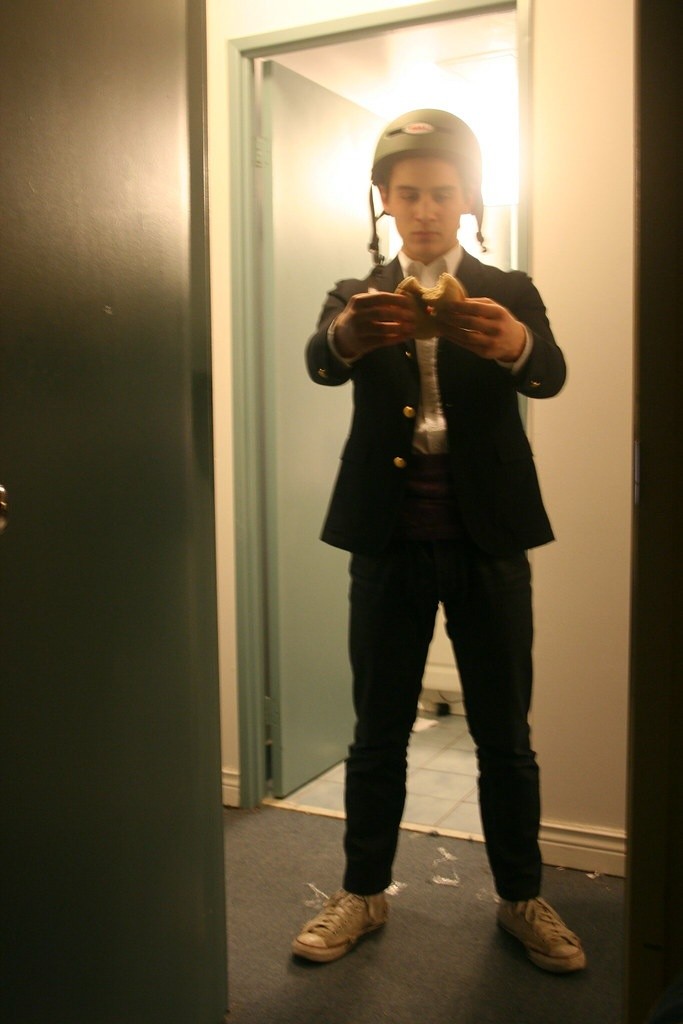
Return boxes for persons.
[289,109,589,973]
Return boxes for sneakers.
[497,896,586,972]
[291,887,386,962]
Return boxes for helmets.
[372,108,483,185]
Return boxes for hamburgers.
[392,273,465,339]
